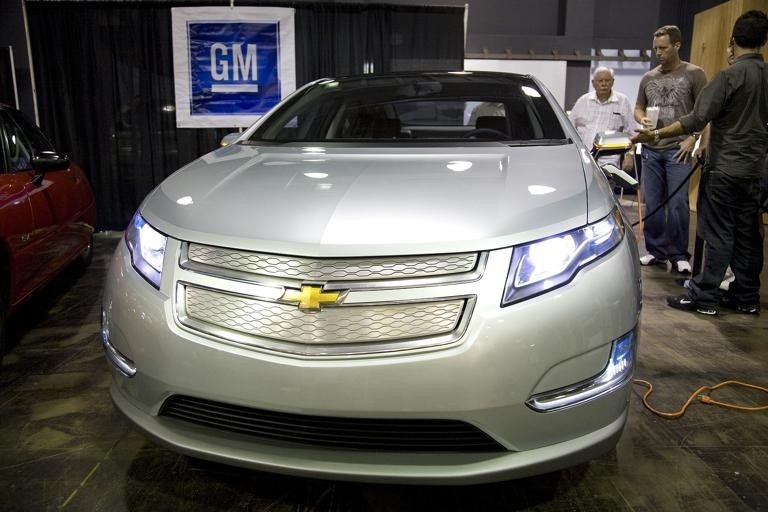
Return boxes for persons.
[627,8,767,318]
[568,65,638,195]
[632,25,707,276]
[670,28,737,298]
[681,32,736,292]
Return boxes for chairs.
[475,116,507,137]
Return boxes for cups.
[646,106,659,130]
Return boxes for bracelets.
[691,133,699,141]
[653,129,659,143]
[625,150,634,156]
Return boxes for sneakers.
[719,276,735,290]
[666,295,719,316]
[640,253,668,265]
[722,297,761,315]
[673,259,691,273]
[684,278,690,288]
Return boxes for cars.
[0,106,98,323]
[101,70,641,486]
